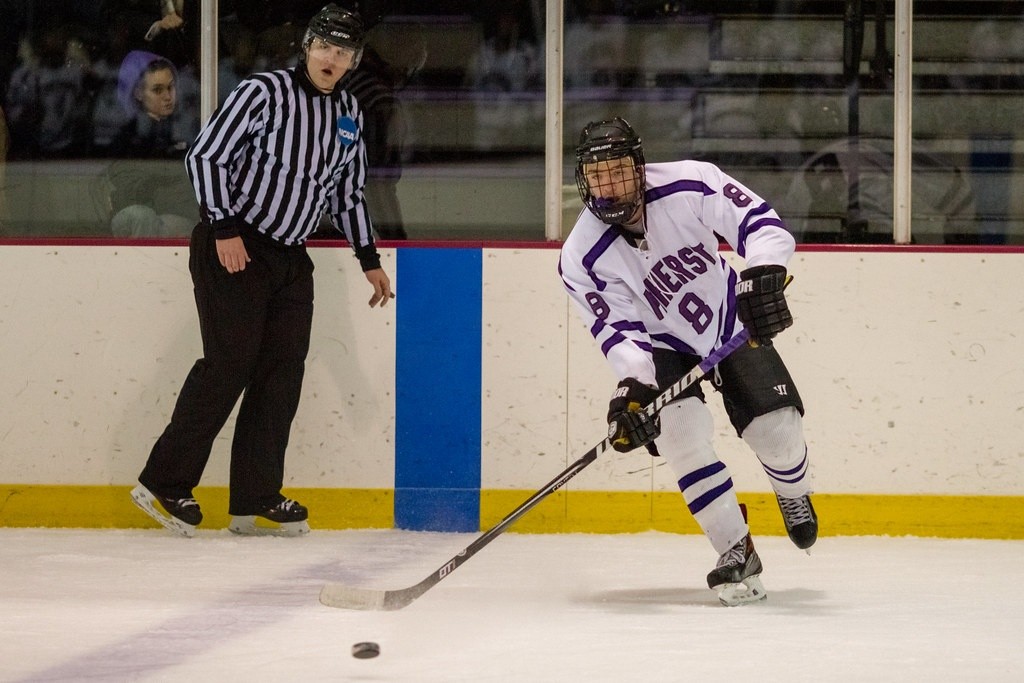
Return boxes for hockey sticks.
[319,330,748,611]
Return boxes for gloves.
[607,378,662,453]
[734,263,793,348]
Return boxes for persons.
[539,115,820,606]
[0,0,647,241]
[131,2,397,535]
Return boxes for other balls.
[351,642,380,659]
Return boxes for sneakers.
[227,493,310,537]
[130,478,202,537]
[706,533,767,606]
[775,490,817,555]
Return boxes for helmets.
[308,3,364,50]
[575,117,646,225]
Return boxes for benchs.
[0,0,1024,247]
